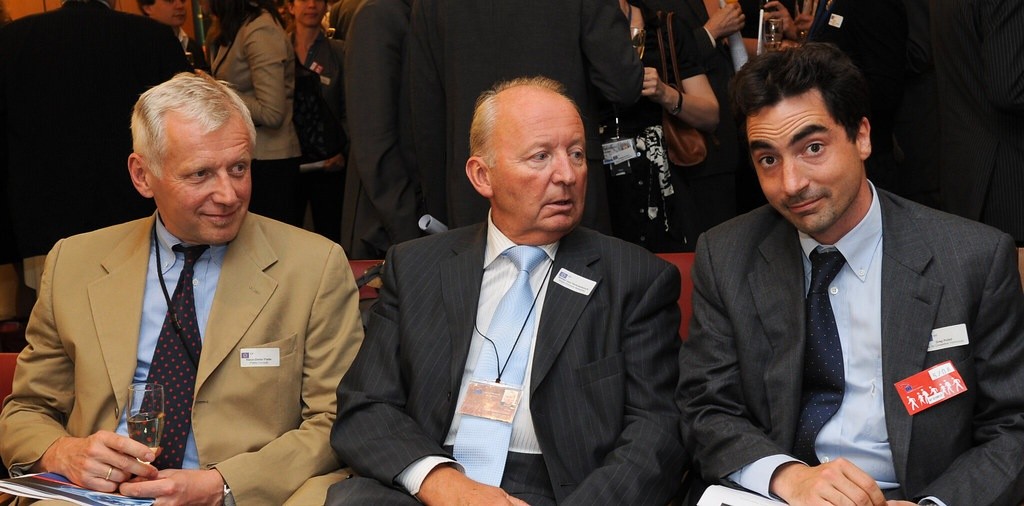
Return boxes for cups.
[630,27,646,60]
[127,383,164,466]
[764,19,784,51]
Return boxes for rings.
[106,466,113,480]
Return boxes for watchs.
[223,482,236,506]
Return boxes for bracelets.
[665,82,683,116]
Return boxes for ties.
[139,243,202,469]
[453,246,547,488]
[790,248,846,466]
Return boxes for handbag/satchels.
[656,10,707,167]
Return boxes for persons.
[615,0,1024,250]
[323,70,689,506]
[344,0,720,253]
[676,48,1024,505]
[0,0,347,350]
[0,71,365,506]
